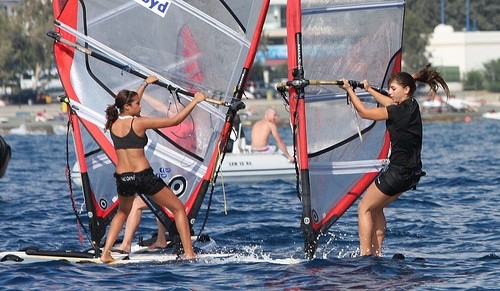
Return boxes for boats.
[67,147,300,188]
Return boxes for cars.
[45,87,65,103]
[11,88,49,106]
[247,80,276,99]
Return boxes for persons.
[251,109,294,163]
[100,74,208,264]
[341,72,427,258]
[111,89,195,254]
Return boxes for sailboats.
[277,0,408,256]
[0,1,270,263]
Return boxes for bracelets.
[143,80,148,85]
[141,82,147,88]
